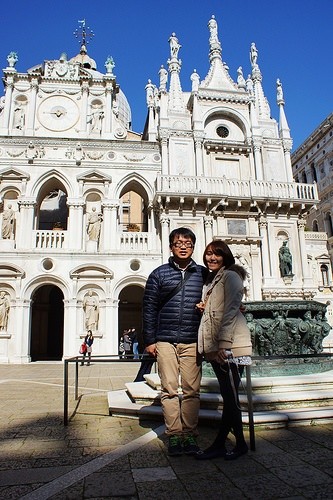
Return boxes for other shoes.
[86,363,90,366]
[225,446,248,457]
[193,448,228,460]
[80,364,84,366]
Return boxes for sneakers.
[167,434,183,455]
[183,434,200,454]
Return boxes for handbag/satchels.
[138,334,145,353]
[79,343,87,354]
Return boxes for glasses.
[171,240,193,248]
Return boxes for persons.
[0,292,10,332]
[248,303,327,357]
[142,228,247,456]
[194,241,254,461]
[81,330,94,366]
[238,257,252,303]
[83,290,98,332]
[0,204,15,240]
[278,239,292,276]
[120,329,139,360]
[86,207,102,241]
[14,14,283,132]
